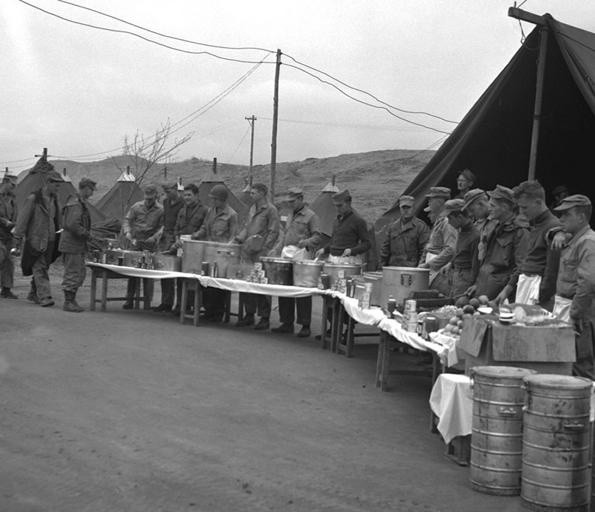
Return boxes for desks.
[181,272,338,353]
[428,373,595,466]
[85,262,180,312]
[339,291,458,434]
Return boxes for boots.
[62,290,85,314]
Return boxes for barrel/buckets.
[291,259,319,288]
[380,265,433,316]
[517,373,594,508]
[321,262,360,295]
[257,256,293,287]
[467,365,536,495]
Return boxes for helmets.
[207,184,229,200]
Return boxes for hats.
[144,184,157,200]
[424,187,453,199]
[330,188,352,206]
[459,188,487,215]
[438,198,468,218]
[79,177,99,191]
[285,188,304,202]
[554,192,593,212]
[398,195,415,209]
[461,167,478,187]
[485,183,517,206]
[162,181,178,190]
[46,171,64,183]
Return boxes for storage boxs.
[455,315,577,377]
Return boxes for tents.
[13,158,108,232]
[307,182,341,238]
[197,170,249,222]
[94,170,145,222]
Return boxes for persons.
[174,183,209,316]
[234,183,280,330]
[418,169,595,381]
[379,196,431,267]
[58,177,98,311]
[315,189,372,346]
[0,174,18,299]
[267,188,321,336]
[153,181,185,312]
[12,172,65,306]
[191,184,238,322]
[122,184,165,310]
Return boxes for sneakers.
[153,303,172,312]
[272,322,294,333]
[122,299,135,309]
[40,297,56,307]
[237,317,255,327]
[27,293,41,304]
[254,319,271,331]
[171,305,192,317]
[297,327,311,338]
[1,290,18,300]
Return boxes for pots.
[323,263,362,288]
[381,266,429,313]
[292,261,323,287]
[260,257,292,286]
